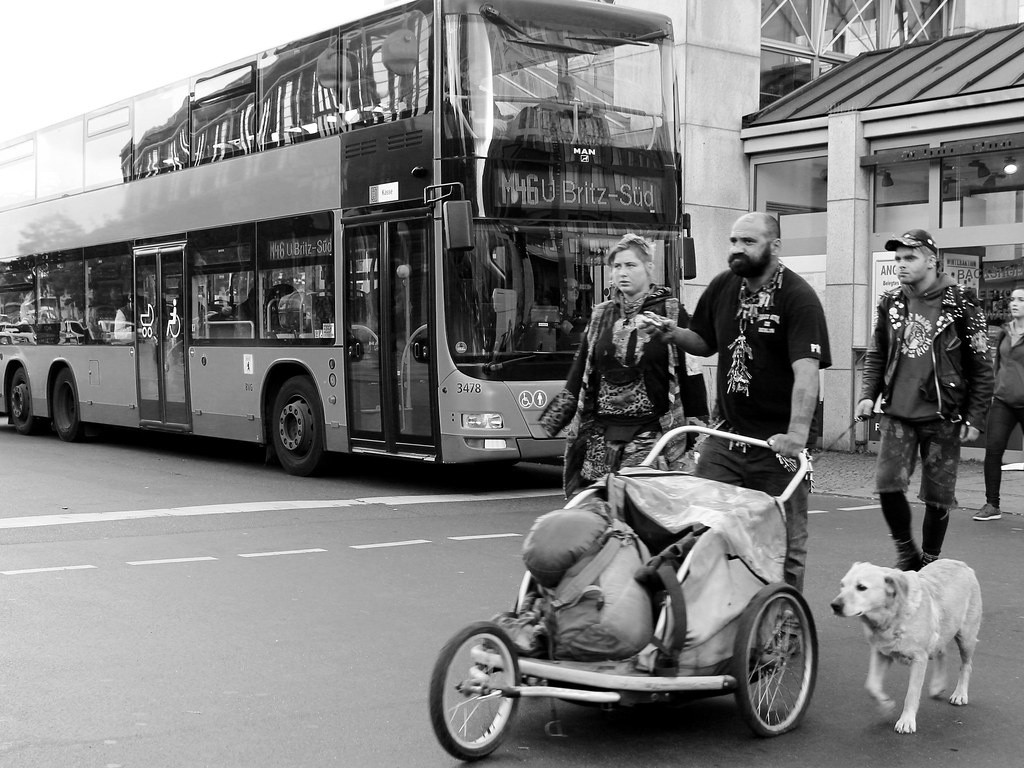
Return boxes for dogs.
[829,558,982,734]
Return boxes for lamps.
[968,160,991,178]
[882,169,894,187]
[1003,156,1017,175]
[983,173,1005,187]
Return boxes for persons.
[556,273,586,332]
[855,229,994,571]
[636,212,832,660]
[973,283,1024,520]
[10,282,305,338]
[534,233,710,504]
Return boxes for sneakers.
[973,503,1002,521]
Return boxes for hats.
[885,229,937,255]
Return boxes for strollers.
[429,423,821,762]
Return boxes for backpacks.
[533,497,651,661]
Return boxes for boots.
[921,551,938,568]
[891,537,920,572]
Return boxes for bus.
[1,0,697,480]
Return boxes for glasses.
[564,286,579,291]
